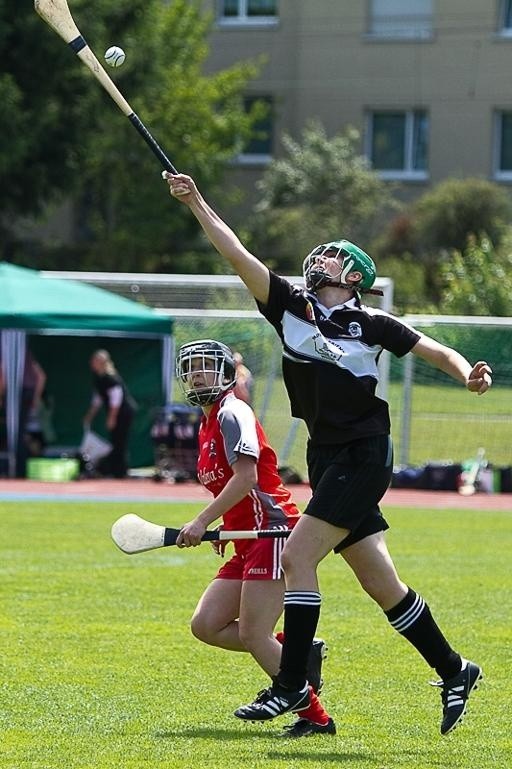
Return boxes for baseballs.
[105,45,125,66]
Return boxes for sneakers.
[282,718,337,737]
[235,677,309,723]
[309,637,328,698]
[441,659,483,735]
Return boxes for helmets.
[303,243,376,291]
[173,340,235,405]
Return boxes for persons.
[0,347,46,479]
[84,349,138,479]
